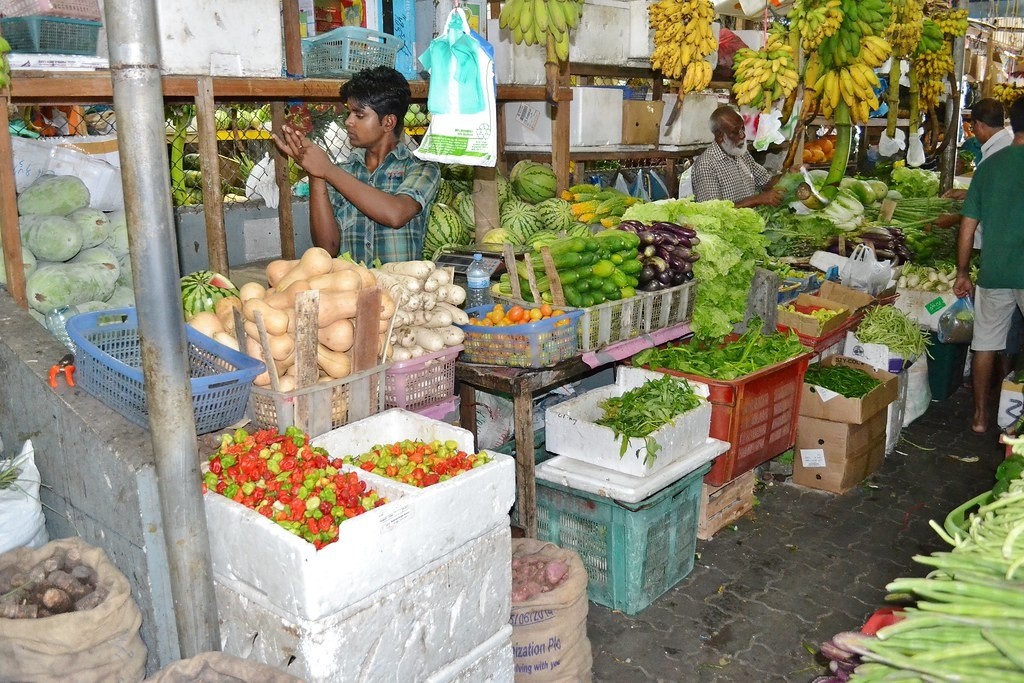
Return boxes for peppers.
[203,426,495,550]
[803,362,881,398]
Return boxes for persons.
[953,94,1024,435]
[693,107,809,208]
[273,64,441,265]
[960,137,982,168]
[935,100,1024,394]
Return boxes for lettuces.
[622,196,772,337]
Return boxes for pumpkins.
[188,246,396,420]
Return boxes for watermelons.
[420,163,594,257]
[179,269,239,321]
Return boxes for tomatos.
[460,305,574,367]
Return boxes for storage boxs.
[486,19,547,86]
[280,0,367,38]
[645,92,718,145]
[378,0,435,79]
[280,26,405,80]
[630,0,657,60]
[0,0,103,56]
[704,22,721,72]
[590,86,648,101]
[568,3,631,68]
[505,86,623,146]
[622,99,665,148]
[731,30,771,54]
[739,97,803,143]
[282,38,308,78]
[66,250,1024,683]
[435,0,488,41]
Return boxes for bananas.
[646,0,719,94]
[992,82,1024,109]
[0,38,13,87]
[731,0,968,125]
[498,0,585,61]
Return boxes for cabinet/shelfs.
[496,60,790,197]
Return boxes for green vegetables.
[992,450,1024,495]
[753,169,842,258]
[592,312,813,468]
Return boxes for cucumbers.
[497,229,644,346]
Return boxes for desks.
[455,313,695,542]
[0,75,503,302]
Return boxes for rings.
[299,146,302,148]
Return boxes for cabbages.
[886,167,960,199]
[790,183,864,231]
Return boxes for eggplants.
[612,219,701,328]
[825,224,912,270]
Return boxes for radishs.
[896,269,958,292]
[368,259,469,402]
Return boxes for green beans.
[854,304,935,368]
[831,487,1024,683]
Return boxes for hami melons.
[800,137,837,163]
